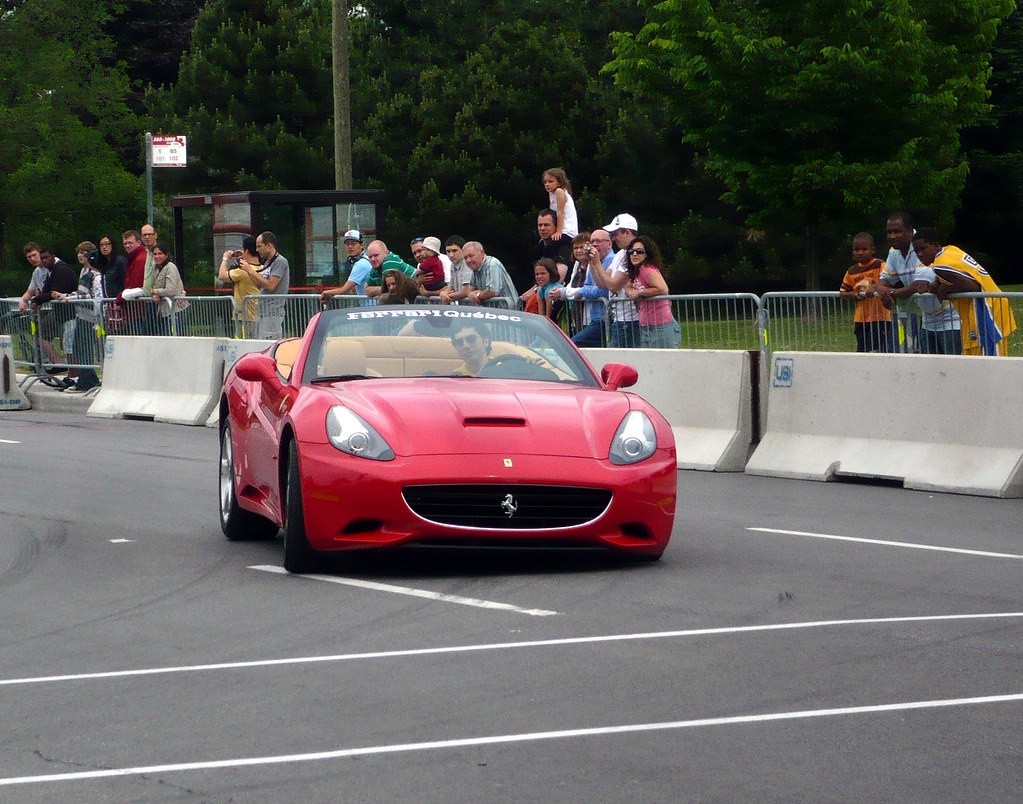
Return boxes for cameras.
[586,246,591,254]
[233,256,243,267]
[232,250,241,258]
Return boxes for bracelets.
[65,292,68,296]
[181,290,186,294]
[477,290,481,301]
[889,288,895,299]
[222,257,229,262]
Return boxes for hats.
[422,237,441,255]
[602,214,638,233]
[342,229,363,243]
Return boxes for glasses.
[411,237,425,244]
[627,249,646,255]
[450,331,478,348]
[100,243,111,247]
[141,233,154,239]
[589,239,611,244]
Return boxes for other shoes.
[65,385,88,393]
[45,367,70,375]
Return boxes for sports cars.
[217,304,678,575]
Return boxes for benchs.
[271,337,574,390]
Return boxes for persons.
[623,234,680,348]
[840,231,886,353]
[542,166,578,284]
[18,241,79,390]
[320,229,377,307]
[365,237,519,310]
[51,223,192,393]
[451,319,546,376]
[219,236,263,339]
[866,211,1017,356]
[518,207,639,347]
[239,230,289,340]
